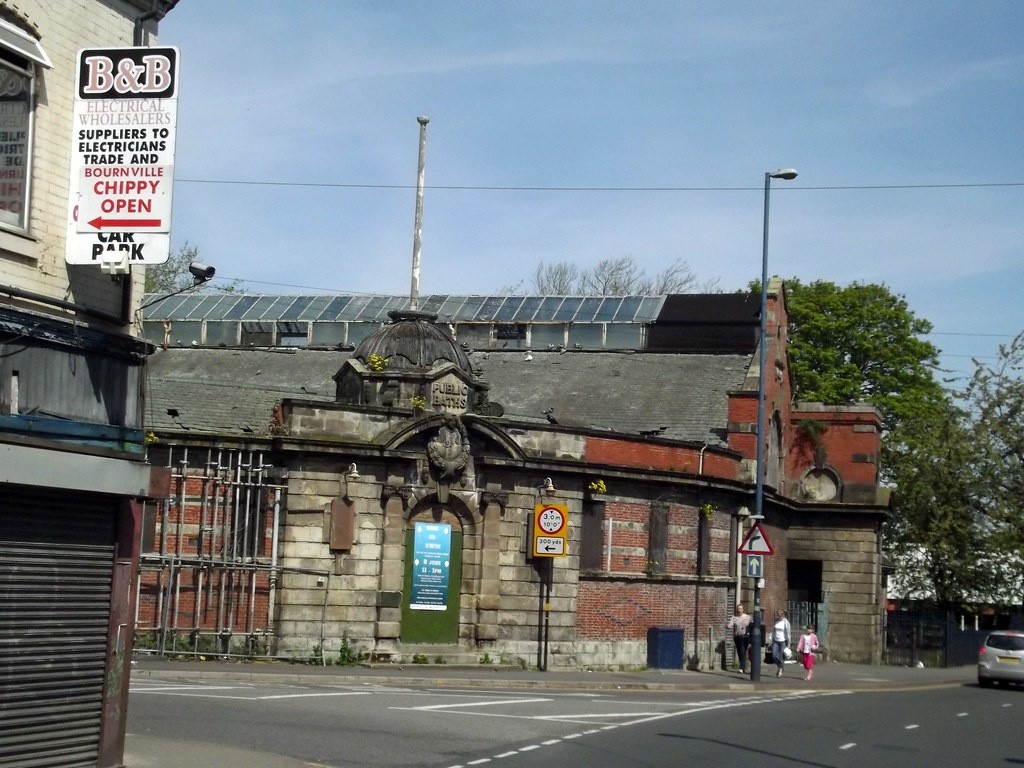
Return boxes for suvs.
[977,630,1024,685]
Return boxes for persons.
[796,624,820,682]
[765,609,791,679]
[725,604,752,673]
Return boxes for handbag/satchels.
[783,648,791,658]
[764,651,785,665]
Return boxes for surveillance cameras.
[189,262,215,277]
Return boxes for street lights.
[752,169,799,682]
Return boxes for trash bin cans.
[646,626,685,670]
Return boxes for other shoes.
[777,675,782,677]
[777,669,784,675]
[738,669,743,674]
